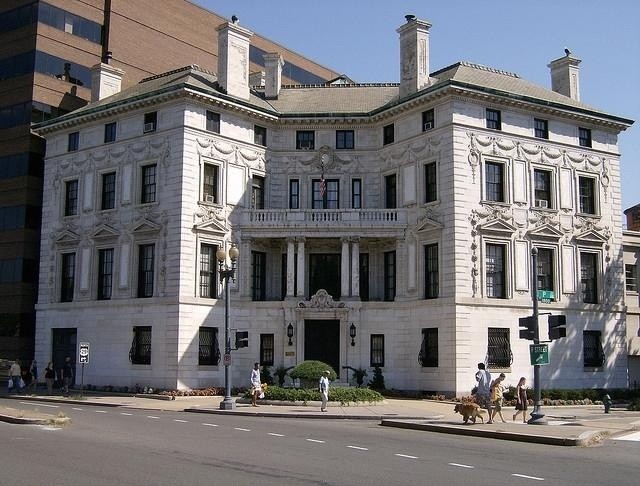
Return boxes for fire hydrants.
[604,393,612,414]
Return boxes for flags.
[319,161,327,198]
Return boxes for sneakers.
[251,401,260,407]
[320,407,328,412]
[470,415,528,424]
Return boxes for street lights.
[216,243,239,410]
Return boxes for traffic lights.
[517,317,533,340]
[550,315,566,339]
[238,330,249,349]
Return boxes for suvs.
[0,352,32,386]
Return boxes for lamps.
[348,322,357,347]
[286,322,294,346]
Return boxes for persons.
[318,369,331,411]
[250,362,262,406]
[513,376,529,423]
[7,357,74,395]
[491,372,506,422]
[470,362,493,423]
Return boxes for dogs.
[454,402,487,424]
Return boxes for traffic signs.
[80,343,89,364]
[530,343,550,366]
